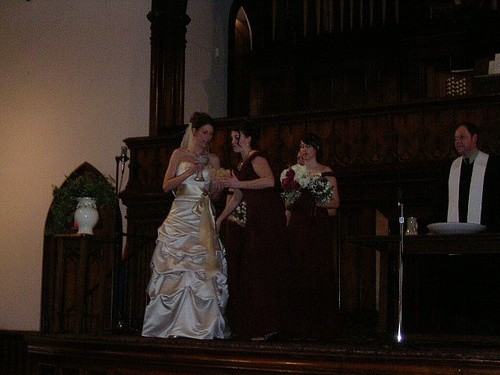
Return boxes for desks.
[360,233,500,335]
[52,233,111,335]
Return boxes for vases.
[75,197,99,234]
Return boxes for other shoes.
[250,330,279,342]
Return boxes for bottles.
[405,216,419,236]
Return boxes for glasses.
[454,135,467,141]
[300,143,313,148]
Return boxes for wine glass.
[194,156,209,181]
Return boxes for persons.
[446,122,500,233]
[142,111,340,340]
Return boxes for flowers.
[231,168,301,226]
[305,172,333,202]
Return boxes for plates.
[426,222,487,235]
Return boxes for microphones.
[121,145,127,173]
